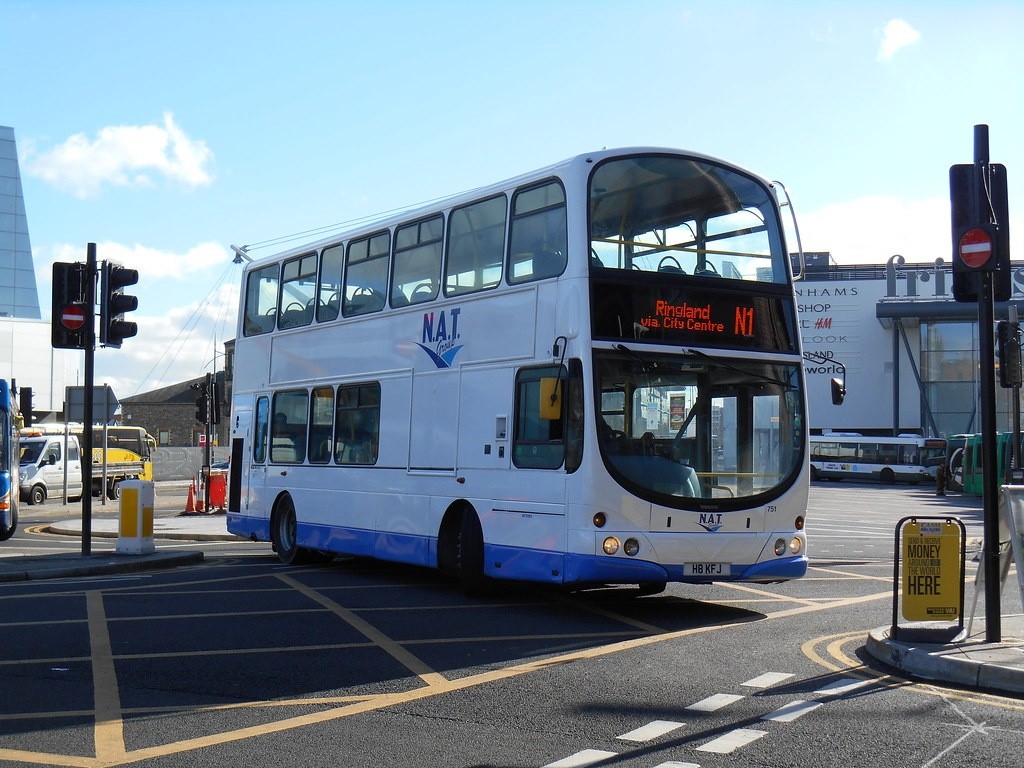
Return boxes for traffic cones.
[182,484,199,515]
[192,474,198,496]
[195,482,205,513]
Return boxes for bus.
[0,379,24,540]
[16,423,158,483]
[809,433,950,486]
[225,143,848,600]
[946,428,1024,497]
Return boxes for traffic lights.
[195,380,210,424]
[99,259,138,348]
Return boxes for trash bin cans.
[210,468,229,510]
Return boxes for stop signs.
[959,229,991,267]
[62,305,86,329]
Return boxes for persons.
[272,413,297,441]
[936,463,947,493]
[544,374,614,444]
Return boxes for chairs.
[304,297,326,324]
[326,292,350,320]
[282,302,305,328]
[410,283,435,303]
[263,307,284,330]
[657,256,687,275]
[351,287,374,315]
[531,242,565,278]
[693,259,721,277]
[591,248,605,268]
[376,283,409,311]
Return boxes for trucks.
[19,434,147,506]
